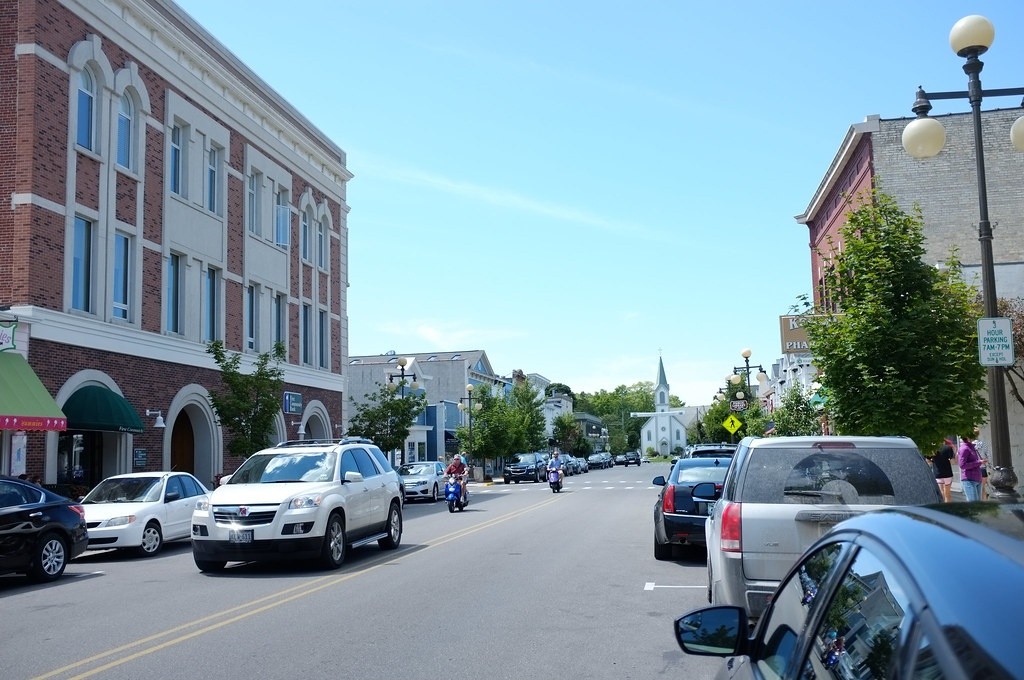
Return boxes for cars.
[396,461,447,502]
[80,471,209,557]
[587,451,641,469]
[540,453,589,477]
[652,458,735,560]
[674,498,1024,680]
[0,475,89,584]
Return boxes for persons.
[821,636,845,670]
[824,461,852,483]
[923,438,955,502]
[443,454,468,503]
[17,448,21,462]
[168,483,182,502]
[33,476,41,488]
[438,455,452,467]
[956,426,988,501]
[460,452,466,464]
[18,474,30,482]
[548,452,564,489]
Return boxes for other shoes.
[460,496,464,503]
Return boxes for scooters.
[823,645,843,670]
[546,467,562,493]
[440,469,470,513]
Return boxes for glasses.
[454,459,459,460]
[554,454,558,455]
[35,481,40,483]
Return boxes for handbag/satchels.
[980,467,988,477]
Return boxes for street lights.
[458,384,483,478]
[588,425,609,451]
[901,15,1024,496]
[730,349,766,434]
[717,374,745,443]
[389,358,419,466]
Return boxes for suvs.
[191,436,401,571]
[504,452,546,484]
[671,444,740,470]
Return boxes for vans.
[689,432,946,607]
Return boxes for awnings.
[61,386,143,436]
[0,352,67,432]
